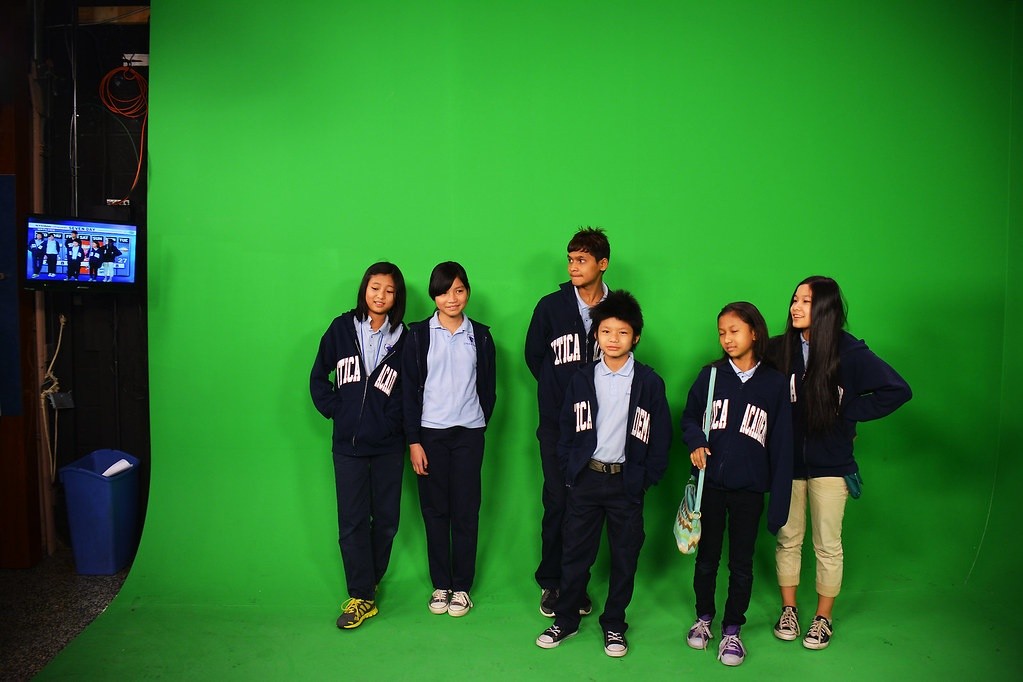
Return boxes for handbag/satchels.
[843,472,864,499]
[673,484,702,555]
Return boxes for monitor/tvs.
[24,213,140,289]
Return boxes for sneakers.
[535,623,578,649]
[428,589,450,614]
[579,592,592,615]
[539,588,560,617]
[603,629,628,657]
[336,597,378,629]
[447,591,473,617]
[802,615,833,650]
[686,614,714,652]
[717,621,747,666]
[773,606,801,641]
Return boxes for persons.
[407,257,498,617]
[44,233,60,277]
[770,276,913,649]
[86,240,102,282]
[310,262,410,630]
[65,231,78,279]
[64,238,85,280]
[681,302,794,666]
[28,232,46,278]
[536,291,674,657]
[102,239,122,282]
[524,227,613,617]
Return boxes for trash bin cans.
[60,448,140,575]
